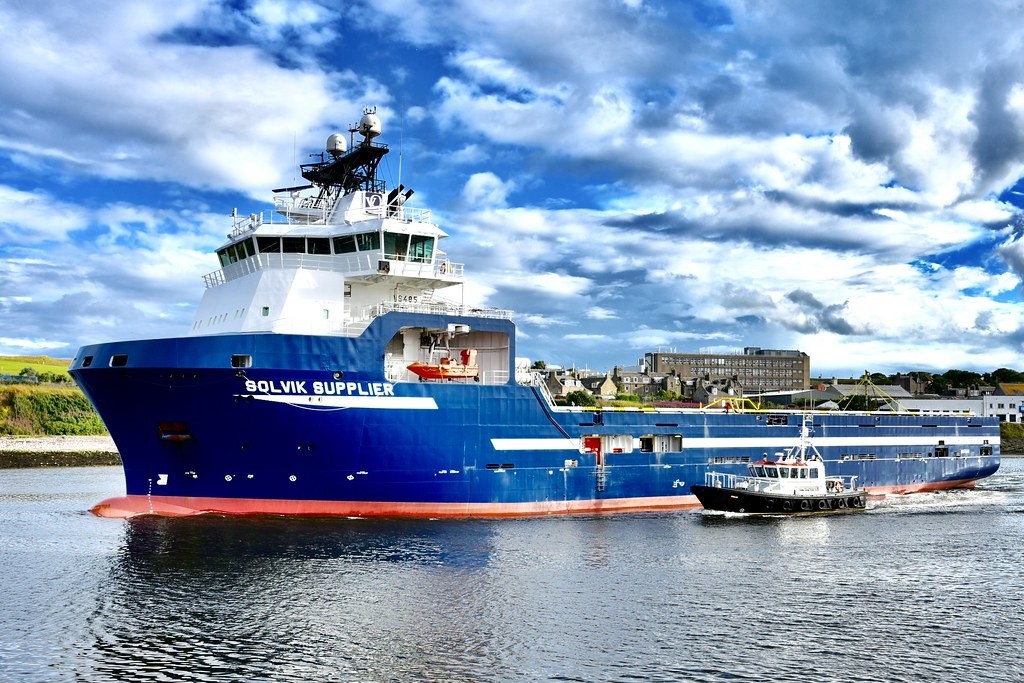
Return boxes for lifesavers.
[439,260,445,275]
[801,500,809,510]
[853,497,860,507]
[837,499,845,509]
[818,500,827,510]
[765,500,775,510]
[835,481,844,492]
[782,501,791,511]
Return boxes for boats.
[405,349,479,378]
[689,392,866,514]
[67,106,1002,518]
